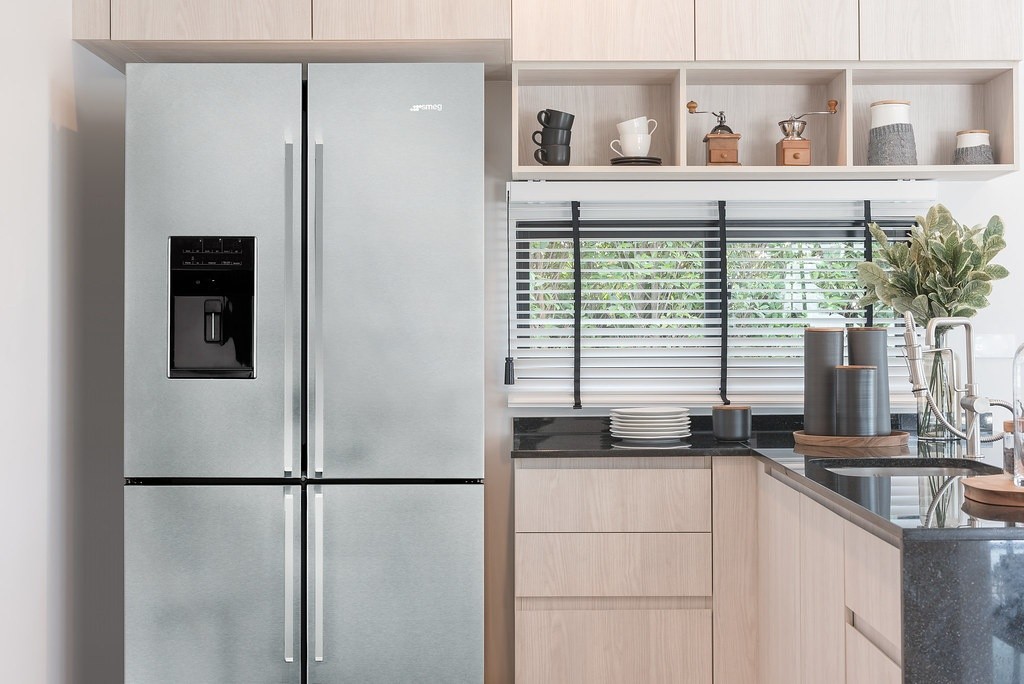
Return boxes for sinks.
[807,448,1005,479]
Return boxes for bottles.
[1002,342,1024,488]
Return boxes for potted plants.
[853,204,1010,441]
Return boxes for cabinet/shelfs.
[72,0,1023,179]
[765,472,904,684]
[514,457,712,684]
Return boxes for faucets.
[924,315,991,457]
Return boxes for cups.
[532,128,571,146]
[534,144,570,166]
[610,134,652,156]
[537,108,575,130]
[616,116,658,135]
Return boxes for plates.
[608,407,692,443]
[610,154,662,166]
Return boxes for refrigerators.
[121,60,486,684]
